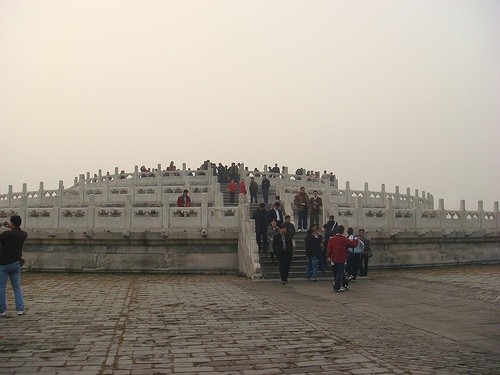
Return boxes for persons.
[358,229,373,276]
[177,189,191,217]
[326,215,338,236]
[272,226,291,285]
[0,215,29,314]
[244,162,284,179]
[252,196,297,263]
[294,186,322,232]
[326,226,360,292]
[188,160,241,183]
[140,166,157,177]
[346,227,363,281]
[295,167,335,186]
[305,223,324,281]
[228,175,270,204]
[106,172,112,180]
[322,225,334,271]
[162,161,181,176]
[94,174,98,182]
[119,170,128,179]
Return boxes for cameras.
[3,222,8,226]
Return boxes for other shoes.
[18,310,26,314]
[336,289,343,292]
[346,284,351,291]
[348,276,354,282]
[0,312,5,316]
[282,281,286,284]
